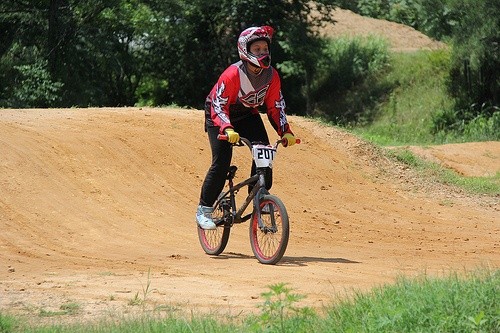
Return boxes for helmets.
[237,26,274,69]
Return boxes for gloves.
[283,132,296,147]
[224,127,240,144]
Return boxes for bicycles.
[196,133,301,265]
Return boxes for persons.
[196,26,296,230]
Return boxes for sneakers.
[253,197,279,213]
[195,204,217,230]
